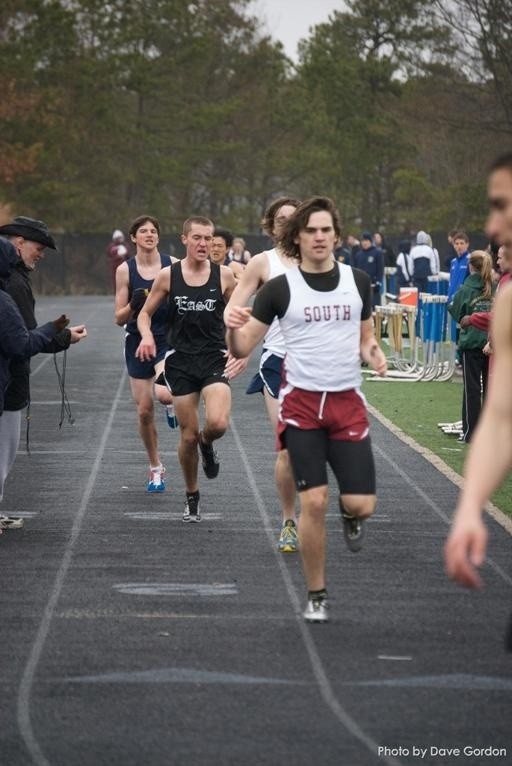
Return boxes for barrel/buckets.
[397,286,419,306]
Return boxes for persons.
[0,215,88,537]
[106,146,512,665]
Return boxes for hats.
[1,216,57,250]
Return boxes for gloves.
[53,315,70,332]
[130,288,145,310]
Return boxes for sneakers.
[1,515,24,530]
